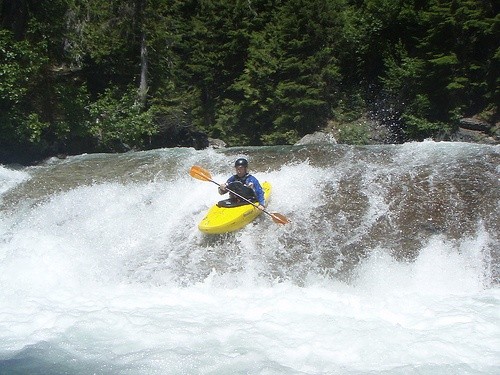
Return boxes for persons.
[218,158,266,211]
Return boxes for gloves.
[220,184,227,192]
[256,205,264,212]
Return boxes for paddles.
[190,165,292,226]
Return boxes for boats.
[198,181,271,234]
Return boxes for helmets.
[235,158,248,168]
